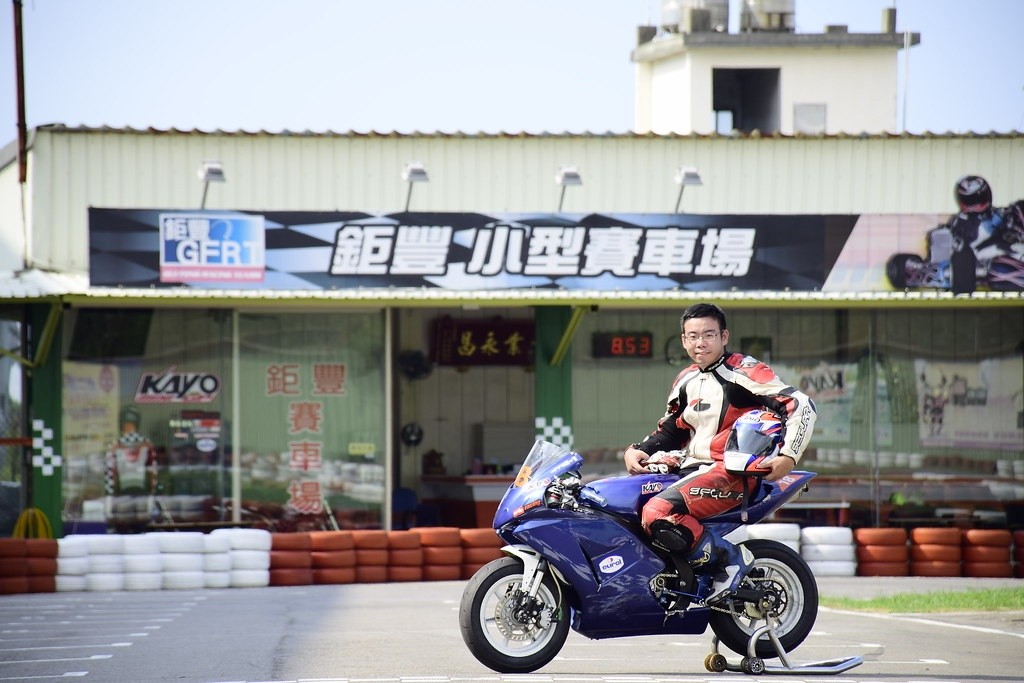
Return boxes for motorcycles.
[459,438,819,673]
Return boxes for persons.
[104,407,156,530]
[624,302,816,605]
[952,176,1010,246]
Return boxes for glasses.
[684,329,724,344]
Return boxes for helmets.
[723,410,787,476]
[955,176,992,222]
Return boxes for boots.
[686,525,755,607]
